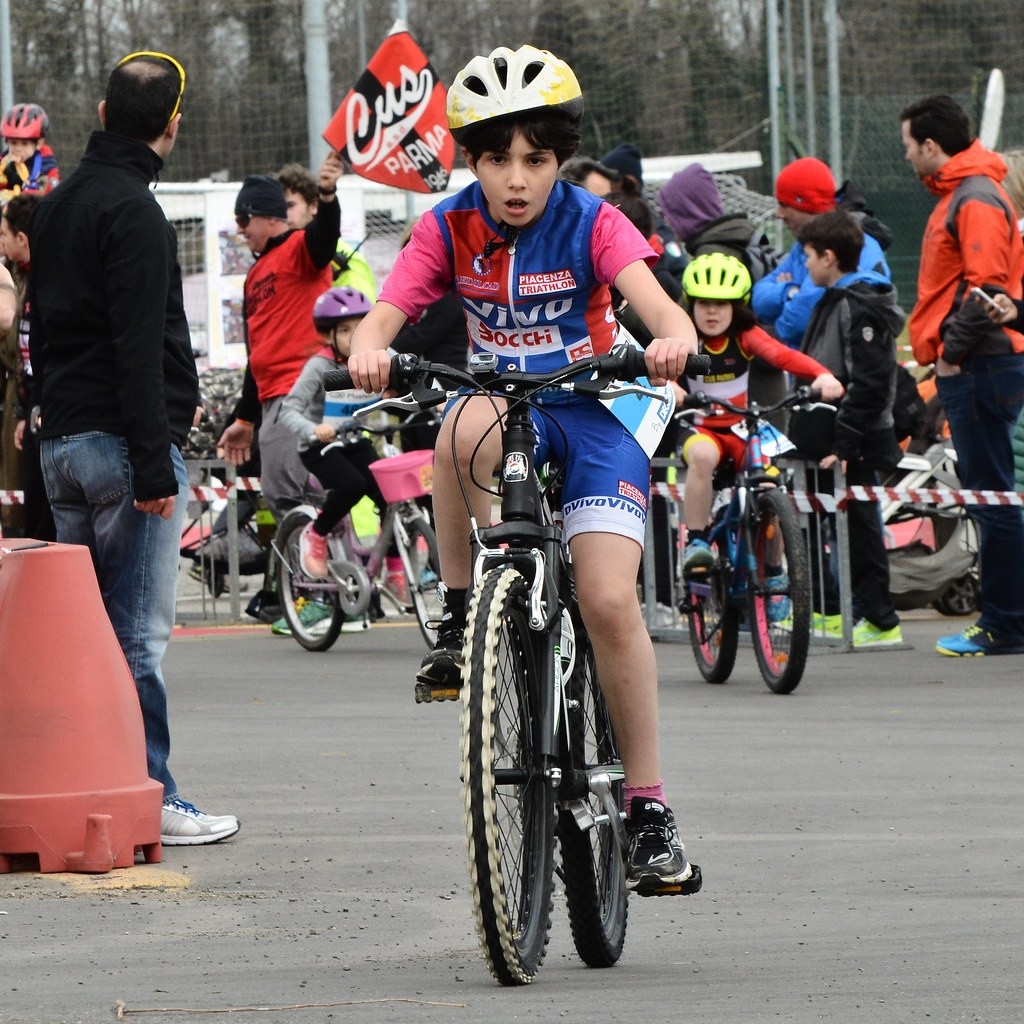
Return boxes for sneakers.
[766,571,793,622]
[160,799,240,845]
[682,538,714,583]
[340,610,371,633]
[772,610,841,638]
[299,520,328,580]
[623,797,693,890]
[852,617,902,646]
[415,610,464,686]
[383,571,414,607]
[271,597,334,635]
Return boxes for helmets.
[680,252,752,305]
[445,44,582,133]
[314,285,373,333]
[0,103,49,138]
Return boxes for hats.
[235,175,288,218]
[777,157,836,214]
[600,144,643,184]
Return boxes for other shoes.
[934,625,1024,656]
[190,558,248,593]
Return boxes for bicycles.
[319,345,717,989]
[263,425,444,652]
[668,386,842,693]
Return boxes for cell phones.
[971,287,1005,316]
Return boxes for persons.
[347,44,699,890]
[899,94,1024,659]
[28,51,241,847]
[558,143,905,646]
[0,104,58,545]
[193,155,446,635]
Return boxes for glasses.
[119,51,185,121]
[236,214,252,227]
